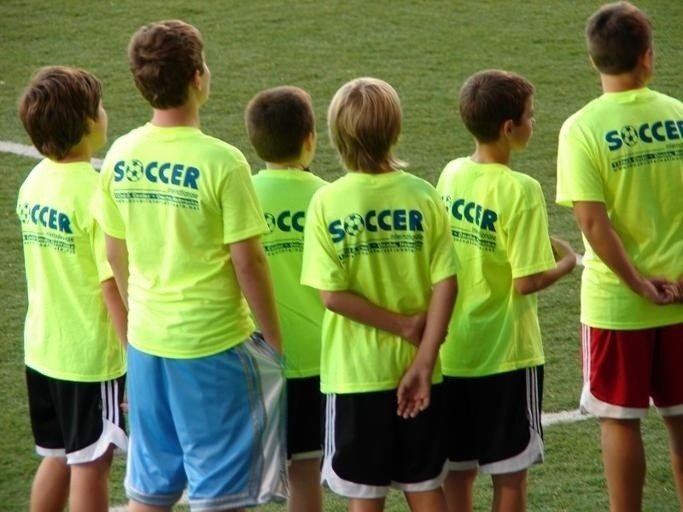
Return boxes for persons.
[298,77,463,510]
[98,18,289,512]
[555,1,683,512]
[246,84,334,510]
[16,63,129,512]
[436,70,578,512]
